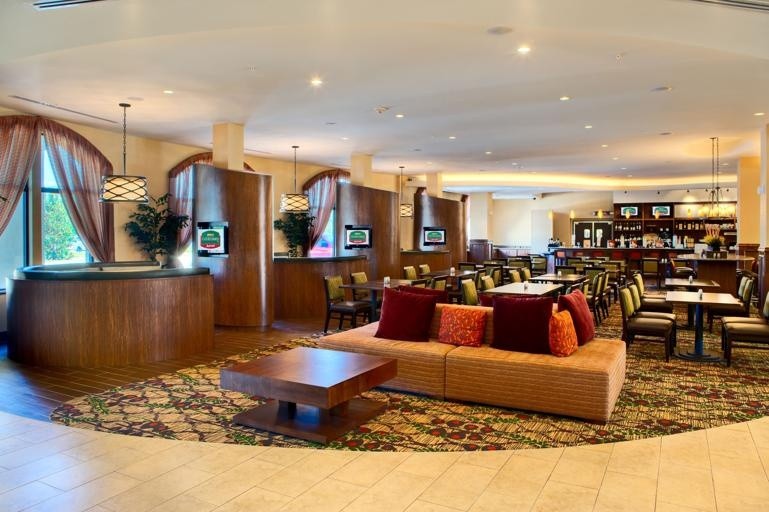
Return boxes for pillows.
[399,286,447,305]
[438,304,486,346]
[374,287,438,344]
[548,311,577,356]
[479,294,535,306]
[491,296,551,354]
[558,290,594,344]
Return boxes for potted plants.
[705,236,724,251]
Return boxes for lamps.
[279,145,310,214]
[687,137,735,225]
[398,166,412,216]
[99,102,148,204]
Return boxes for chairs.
[532,258,547,283]
[323,277,371,334]
[618,286,673,361]
[641,258,662,289]
[587,272,608,317]
[480,275,495,292]
[609,260,630,280]
[510,260,532,278]
[410,279,427,289]
[572,263,593,273]
[474,270,484,291]
[632,274,673,314]
[485,265,503,286]
[520,267,533,282]
[707,277,750,326]
[724,323,769,369]
[404,267,418,281]
[736,262,760,292]
[586,274,602,326]
[720,291,769,353]
[669,259,695,279]
[461,280,478,307]
[456,263,477,289]
[587,259,605,266]
[425,275,448,289]
[584,267,611,306]
[493,259,509,281]
[708,278,754,335]
[567,257,581,266]
[599,262,623,301]
[419,265,431,280]
[554,266,577,275]
[627,282,677,352]
[489,268,499,286]
[352,273,387,326]
[509,270,521,283]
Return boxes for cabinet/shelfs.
[612,218,644,247]
[645,219,675,247]
[676,218,737,246]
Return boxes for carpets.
[51,328,765,452]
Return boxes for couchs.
[319,303,626,422]
